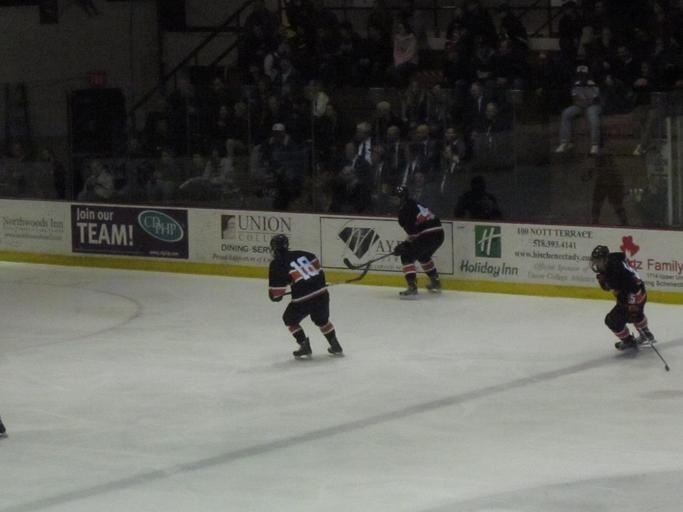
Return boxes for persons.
[390,183,445,295]
[1,0,682,229]
[591,245,654,349]
[267,234,343,355]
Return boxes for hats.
[271,124,286,132]
[577,66,588,73]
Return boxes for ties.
[360,143,367,158]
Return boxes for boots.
[614,328,654,350]
[327,337,343,354]
[399,278,418,296]
[292,336,312,357]
[424,272,442,290]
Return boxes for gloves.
[393,243,405,256]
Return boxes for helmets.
[269,235,288,250]
[590,245,609,261]
[390,186,408,200]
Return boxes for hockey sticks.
[634,322,670,371]
[283,260,371,296]
[342,253,394,269]
